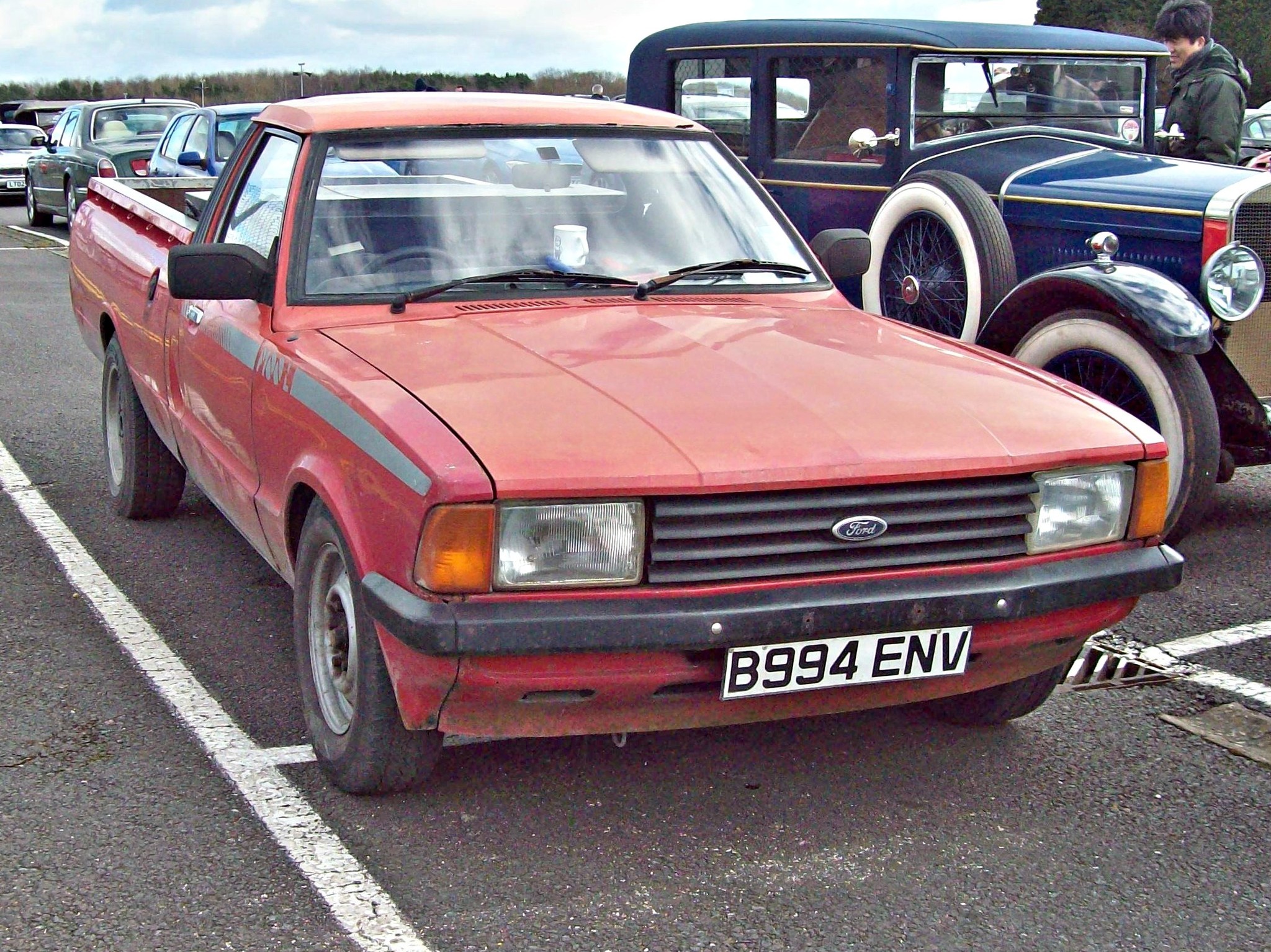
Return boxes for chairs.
[305,254,397,293]
[218,131,236,157]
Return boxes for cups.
[553,223,589,267]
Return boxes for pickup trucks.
[67,92,1181,799]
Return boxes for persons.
[972,65,1114,135]
[454,86,465,91]
[590,84,604,100]
[1155,0,1251,167]
[415,78,435,92]
[1078,66,1124,137]
[790,60,942,163]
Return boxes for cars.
[0,98,399,235]
[625,18,1271,538]
[1120,102,1271,170]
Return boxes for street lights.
[195,79,211,106]
[123,93,128,99]
[292,63,311,99]
